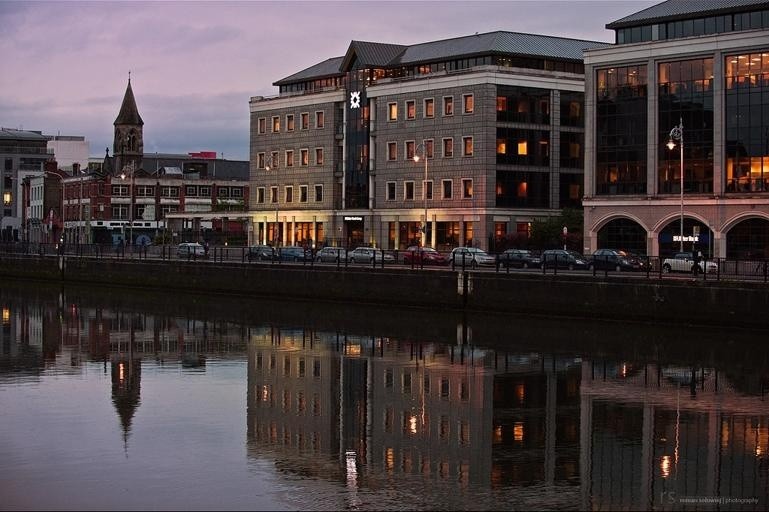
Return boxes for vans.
[176,242,210,259]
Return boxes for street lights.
[664,115,686,251]
[120,158,135,242]
[412,143,428,248]
